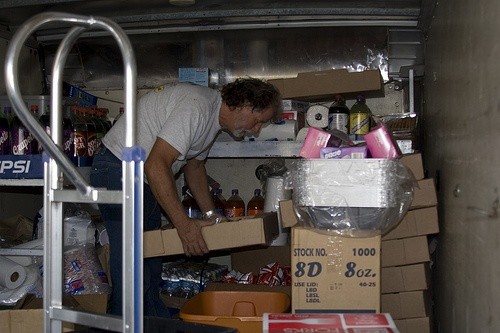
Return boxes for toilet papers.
[0,257,26,291]
[261,175,291,247]
[306,104,332,128]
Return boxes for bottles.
[181,197,204,218]
[213,189,227,216]
[328,96,349,134]
[0,104,125,167]
[247,189,263,217]
[349,96,372,134]
[227,190,245,221]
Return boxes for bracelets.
[201,210,218,220]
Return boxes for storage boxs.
[0,127,440,333]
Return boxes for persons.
[90,77,283,333]
